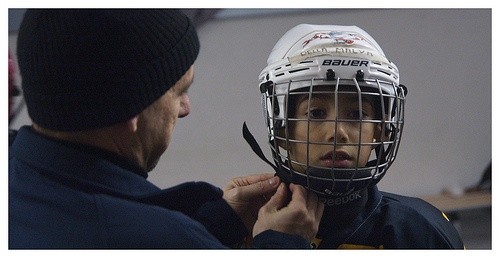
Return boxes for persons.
[8,8,325,249]
[239,22,465,249]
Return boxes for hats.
[17,8,199,131]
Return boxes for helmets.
[258,23,401,128]
